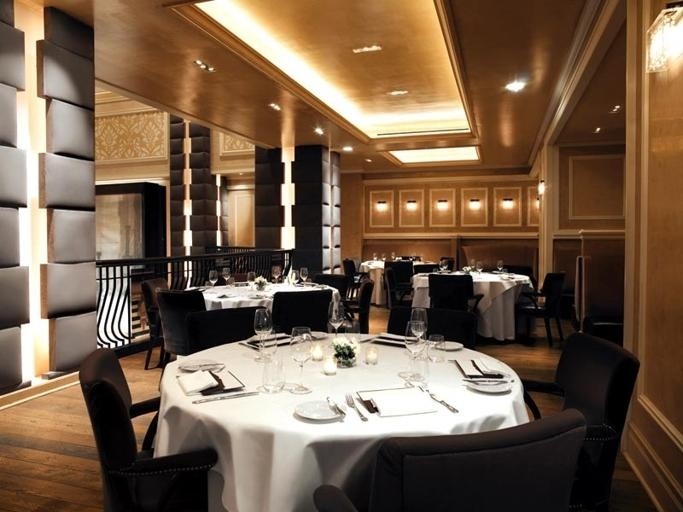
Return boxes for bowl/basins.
[471,370,511,389]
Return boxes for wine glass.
[438,257,506,279]
[206,265,310,297]
[369,249,427,261]
[251,307,312,395]
[404,308,444,384]
[325,299,346,336]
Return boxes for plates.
[177,356,225,373]
[434,341,463,350]
[465,385,513,394]
[296,400,344,420]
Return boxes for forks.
[344,395,368,422]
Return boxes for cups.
[363,345,380,366]
[311,341,326,360]
[323,356,337,377]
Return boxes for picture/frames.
[558,144,624,231]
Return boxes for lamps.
[644,6,683,73]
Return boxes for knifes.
[419,385,459,414]
[326,396,346,417]
[462,378,515,384]
[192,391,260,405]
